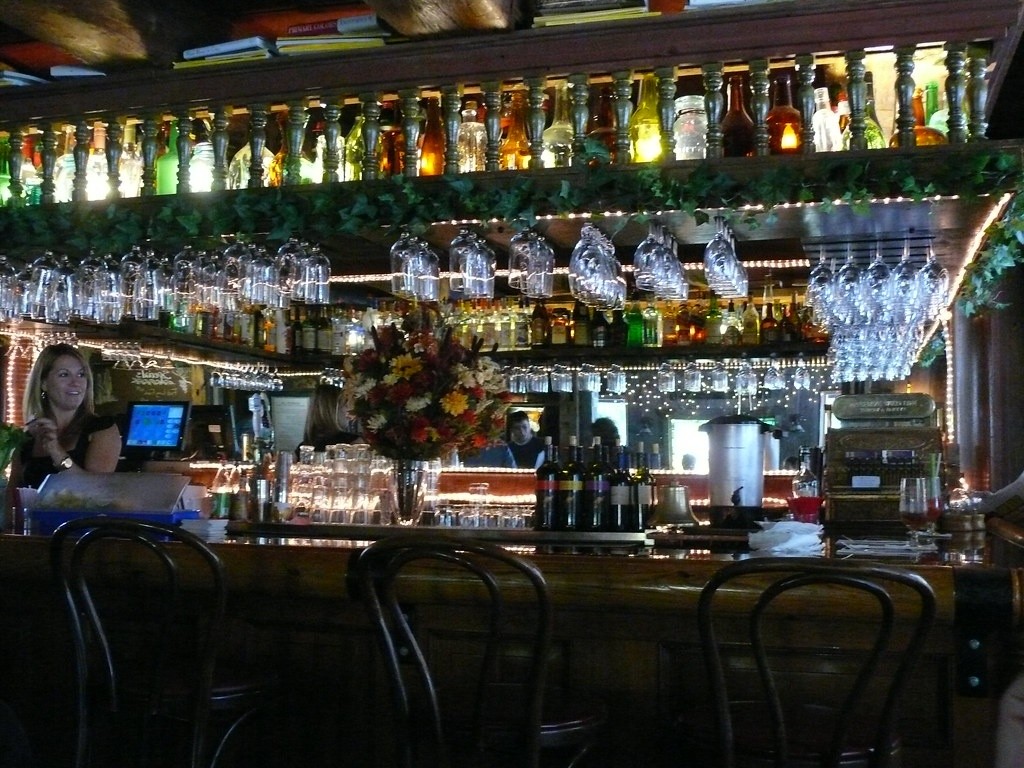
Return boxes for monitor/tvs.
[123,401,192,461]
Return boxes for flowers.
[339,296,513,519]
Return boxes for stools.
[43,513,287,768]
[354,533,611,768]
[675,557,938,768]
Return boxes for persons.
[507,411,544,468]
[3,344,122,526]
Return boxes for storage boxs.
[26,470,201,542]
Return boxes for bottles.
[287,299,826,357]
[163,294,280,352]
[537,435,656,533]
[293,444,528,529]
[0,68,974,206]
[793,451,819,497]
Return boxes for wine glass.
[40,331,349,393]
[925,477,944,536]
[0,239,332,326]
[899,478,928,548]
[498,360,813,396]
[810,245,947,382]
[391,215,753,305]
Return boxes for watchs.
[50,457,73,474]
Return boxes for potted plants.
[0,422,32,532]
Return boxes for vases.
[386,459,430,525]
